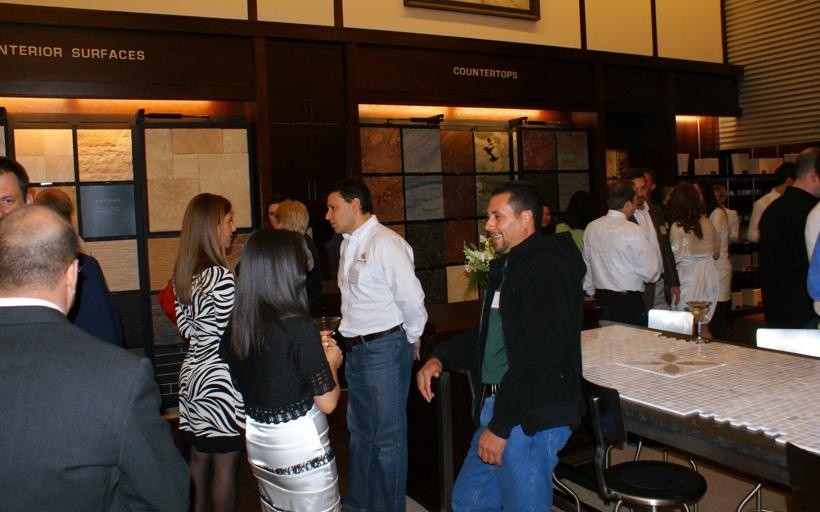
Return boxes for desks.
[581,324,820,511]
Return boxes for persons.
[541,167,680,329]
[272,195,324,318]
[415,180,589,511]
[664,174,740,339]
[747,147,818,328]
[217,229,347,512]
[325,177,431,511]
[0,203,193,510]
[236,186,329,282]
[0,157,127,351]
[172,191,248,510]
[32,188,88,258]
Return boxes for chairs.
[756,328,820,358]
[466,367,592,511]
[647,308,696,338]
[579,376,707,512]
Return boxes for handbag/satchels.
[156,277,177,324]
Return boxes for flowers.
[463,234,498,289]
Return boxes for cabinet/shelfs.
[682,173,776,317]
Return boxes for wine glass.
[312,315,343,350]
[686,299,711,344]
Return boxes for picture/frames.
[403,0,539,22]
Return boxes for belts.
[351,325,401,345]
[481,383,499,397]
